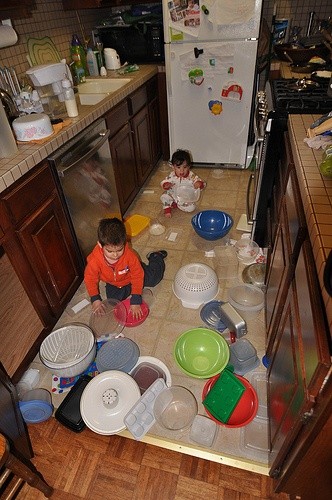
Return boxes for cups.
[14,361,54,423]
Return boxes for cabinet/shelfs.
[4,160,86,313]
[262,129,332,495]
[104,73,161,218]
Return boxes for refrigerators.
[162,0,263,170]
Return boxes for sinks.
[79,94,109,105]
[73,78,132,92]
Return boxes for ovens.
[245,100,288,248]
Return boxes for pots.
[289,62,330,73]
[272,43,321,63]
[311,70,332,82]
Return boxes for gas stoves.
[256,78,332,134]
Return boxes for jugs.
[104,48,121,70]
[25,58,73,121]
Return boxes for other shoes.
[147,250,167,260]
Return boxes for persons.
[160,150,206,219]
[84,217,167,321]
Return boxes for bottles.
[0,98,18,158]
[63,75,79,118]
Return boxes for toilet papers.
[0,24,18,50]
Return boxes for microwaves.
[98,22,165,64]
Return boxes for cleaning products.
[86,48,99,76]
[70,34,88,77]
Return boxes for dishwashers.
[45,118,122,269]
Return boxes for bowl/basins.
[153,386,198,433]
[12,114,55,142]
[234,239,260,264]
[202,374,259,428]
[39,322,96,378]
[173,327,230,379]
[190,210,234,242]
[226,283,264,321]
[242,263,265,292]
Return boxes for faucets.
[68,59,76,66]
